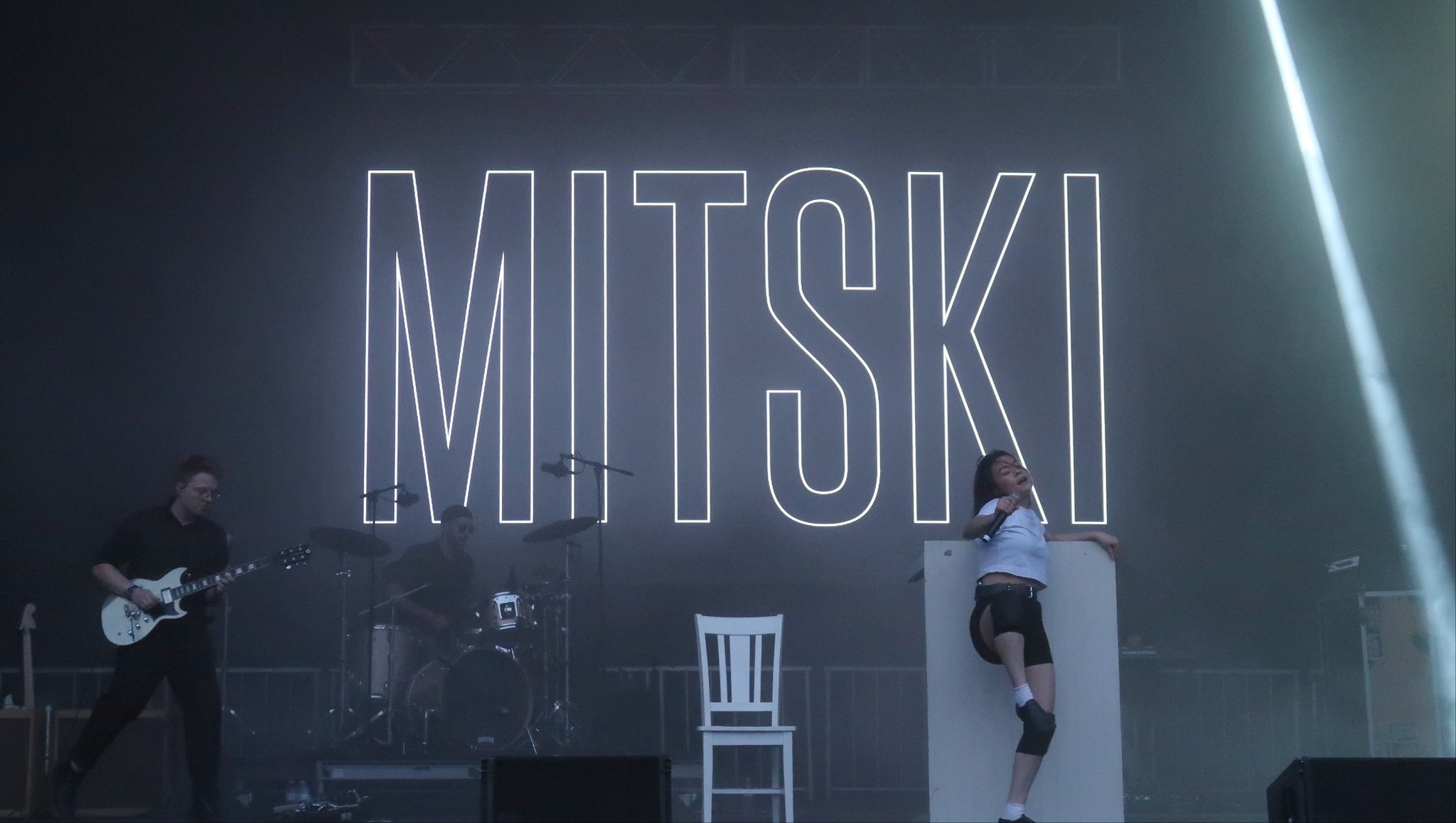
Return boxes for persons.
[963,449,1120,823]
[380,503,476,761]
[39,455,238,823]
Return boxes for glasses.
[458,523,478,536]
[185,484,222,499]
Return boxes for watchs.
[126,584,138,601]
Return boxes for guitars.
[4,603,51,776]
[100,541,313,646]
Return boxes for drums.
[450,626,515,656]
[480,589,541,646]
[405,647,533,750]
[521,577,574,604]
[352,623,426,715]
[534,699,588,747]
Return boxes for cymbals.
[522,516,599,542]
[309,527,391,556]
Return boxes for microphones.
[395,493,419,507]
[983,492,1023,543]
[540,461,573,476]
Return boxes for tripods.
[341,578,444,752]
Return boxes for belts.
[974,582,1038,599]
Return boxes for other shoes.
[45,763,79,822]
[186,799,222,823]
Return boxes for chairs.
[691,610,800,823]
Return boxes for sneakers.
[1016,698,1056,734]
[997,813,1035,823]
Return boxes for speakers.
[0,708,34,819]
[222,668,322,809]
[480,757,670,822]
[54,708,167,818]
[321,766,474,822]
